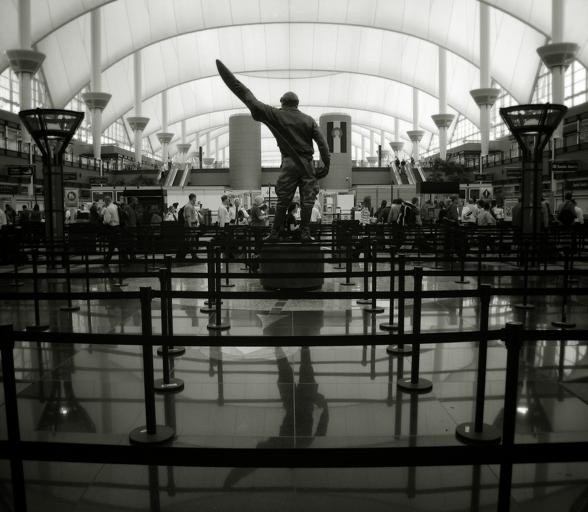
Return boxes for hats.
[255,197,263,205]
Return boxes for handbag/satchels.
[439,217,456,227]
[556,201,573,225]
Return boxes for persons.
[1,189,205,271]
[234,81,331,245]
[400,159,406,174]
[360,189,587,270]
[254,345,330,451]
[394,158,400,173]
[216,192,322,228]
